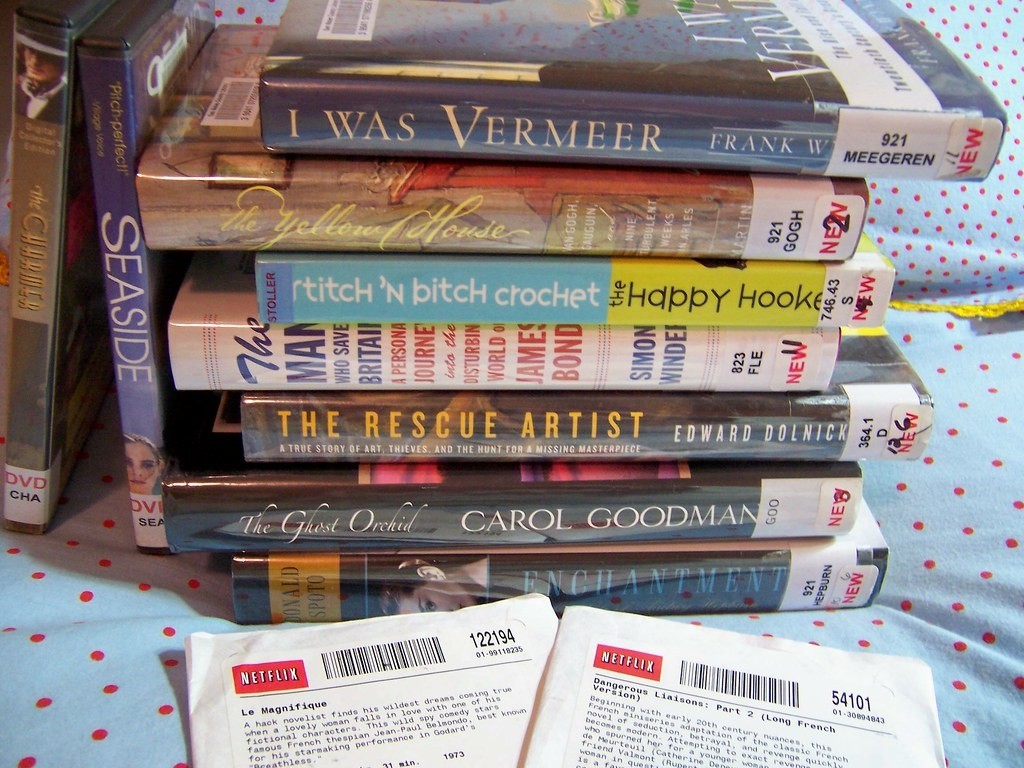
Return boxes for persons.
[122,433,165,496]
[380,558,487,616]
[15,46,66,125]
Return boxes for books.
[161,397,861,555]
[75,0,216,556]
[231,494,890,626]
[168,250,842,391]
[255,232,896,328]
[261,0,1003,183]
[522,605,948,768]
[241,329,935,462]
[0,0,77,536]
[136,23,870,261]
[186,593,558,767]
